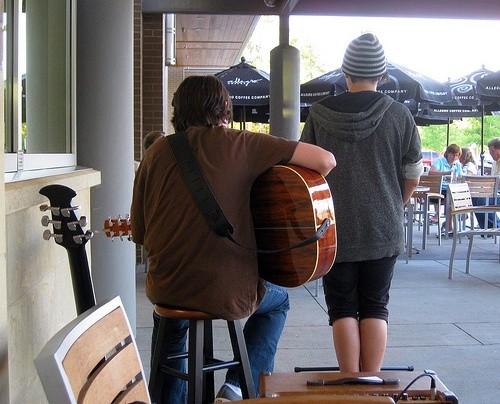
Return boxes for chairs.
[404,175,500,280]
[32,294,152,404]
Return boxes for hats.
[342,33,387,78]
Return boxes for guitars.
[103,162,336,287]
[39,185,106,378]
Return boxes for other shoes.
[215,383,242,404]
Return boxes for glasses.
[490,149,495,154]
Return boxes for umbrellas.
[215,56,313,131]
[300,56,500,176]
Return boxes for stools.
[148,304,257,404]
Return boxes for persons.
[428,138,500,238]
[299,31,424,372]
[143,131,166,150]
[129,73,337,404]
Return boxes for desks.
[404,186,431,254]
